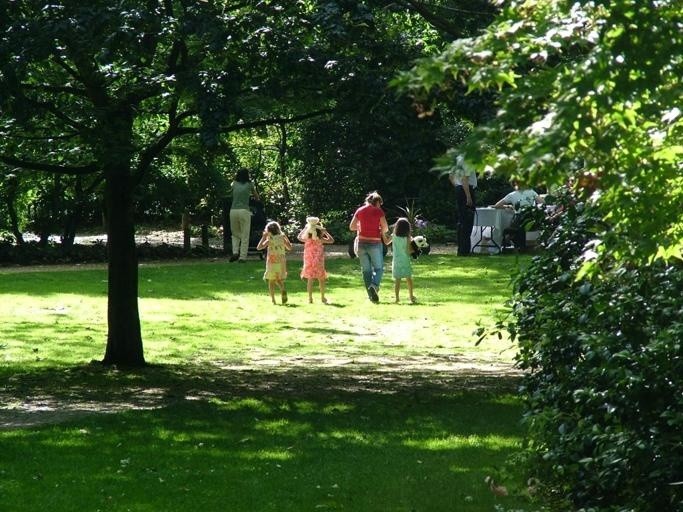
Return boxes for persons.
[492,177,545,254]
[255,221,293,305]
[446,143,480,257]
[348,190,389,306]
[226,166,259,266]
[296,216,335,304]
[379,217,419,305]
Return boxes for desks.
[471,204,554,255]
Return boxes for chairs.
[503,202,539,254]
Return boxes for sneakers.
[229,252,245,264]
[368,284,379,303]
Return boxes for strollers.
[248,197,268,259]
